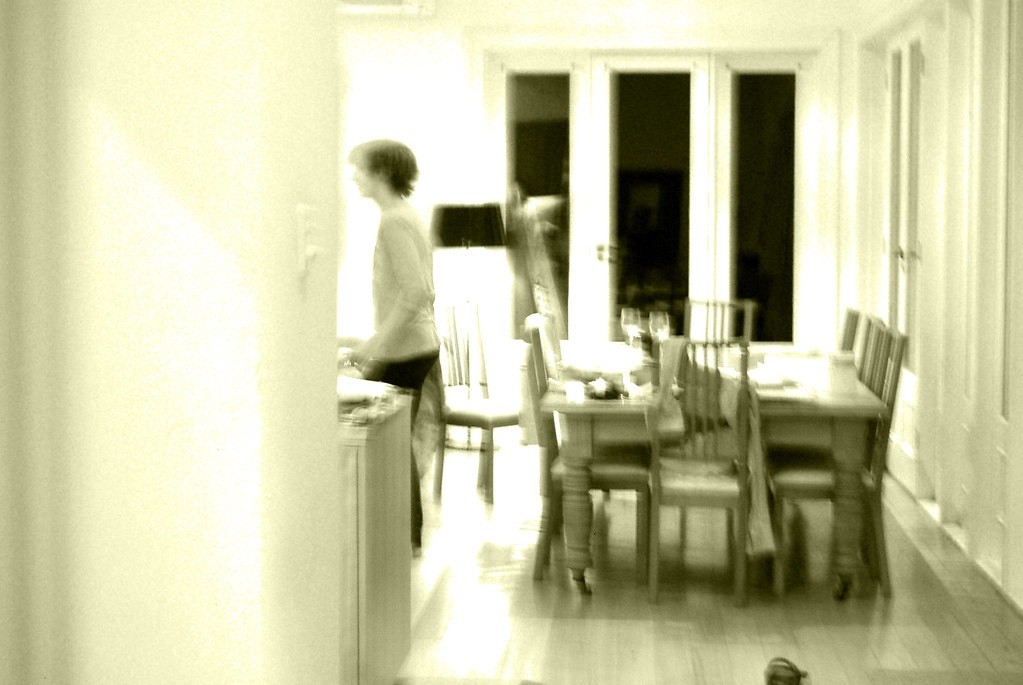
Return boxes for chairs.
[431,299,907,605]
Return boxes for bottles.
[828,350,859,403]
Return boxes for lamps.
[431,203,508,249]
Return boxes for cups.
[648,311,670,339]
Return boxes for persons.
[347,139,437,560]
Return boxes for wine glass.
[619,307,642,348]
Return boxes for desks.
[541,340,889,601]
